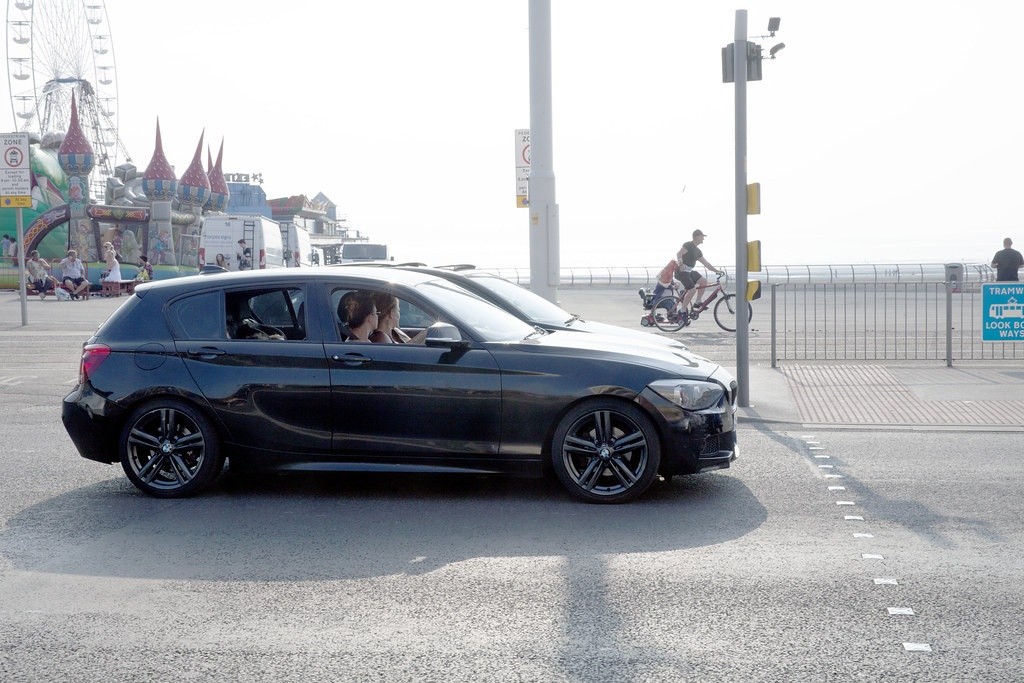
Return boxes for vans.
[340,243,394,263]
[281,221,319,269]
[198,215,287,274]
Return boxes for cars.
[61,263,740,506]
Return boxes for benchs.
[16,282,92,301]
[101,280,153,297]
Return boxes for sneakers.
[693,302,708,310]
[678,310,687,321]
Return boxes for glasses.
[369,311,381,316]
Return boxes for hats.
[693,229,707,237]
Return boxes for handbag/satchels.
[115,253,123,263]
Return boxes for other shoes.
[70,292,80,301]
[39,291,46,300]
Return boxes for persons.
[215,254,226,268]
[673,229,725,321]
[127,254,154,295]
[103,250,121,295]
[369,289,430,345]
[59,250,89,300]
[101,241,118,277]
[8,237,17,256]
[338,293,381,344]
[26,250,53,299]
[991,237,1024,282]
[233,238,251,273]
[0,233,10,263]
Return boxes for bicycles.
[651,272,753,333]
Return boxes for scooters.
[639,282,692,327]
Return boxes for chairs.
[297,300,308,341]
